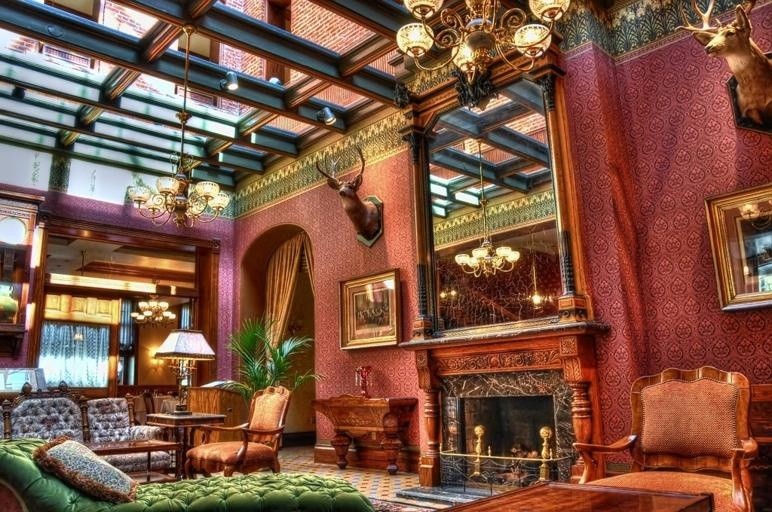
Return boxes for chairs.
[573,366,759,512]
[184,386,292,479]
[143,391,155,421]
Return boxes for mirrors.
[395,41,596,339]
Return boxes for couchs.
[0,381,171,475]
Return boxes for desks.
[310,396,417,475]
[145,413,227,480]
[133,394,171,412]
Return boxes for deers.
[674,0,772,125]
[316,144,380,242]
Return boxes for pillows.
[32,436,138,504]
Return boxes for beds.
[0,437,376,511]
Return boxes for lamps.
[127,25,230,229]
[454,138,521,278]
[396,0,571,85]
[530,231,542,304]
[317,107,337,126]
[220,71,238,91]
[130,294,177,328]
[154,329,216,416]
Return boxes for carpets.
[367,497,438,512]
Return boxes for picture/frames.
[338,267,403,350]
[702,180,772,312]
